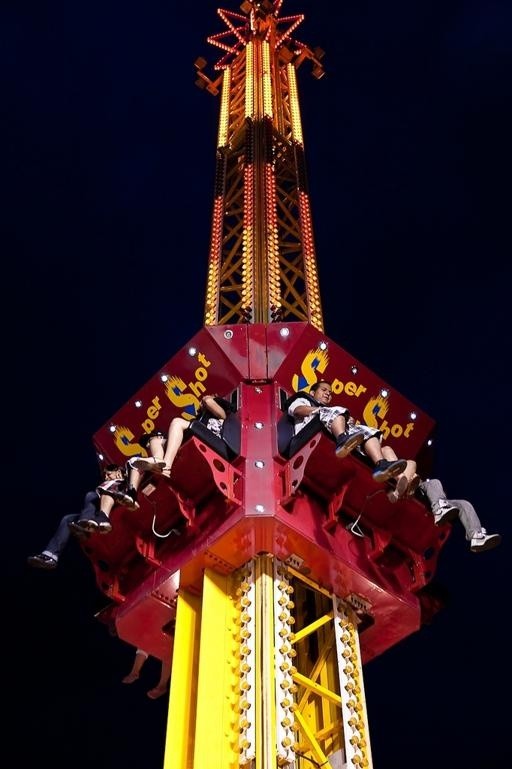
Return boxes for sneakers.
[131,456,172,479]
[470,527,501,552]
[28,554,57,570]
[371,460,407,483]
[408,474,420,496]
[388,476,408,504]
[434,499,460,527]
[115,488,140,512]
[335,431,364,458]
[67,511,112,540]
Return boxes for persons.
[381,445,420,505]
[288,380,408,484]
[77,429,165,535]
[132,394,233,479]
[27,464,124,569]
[419,478,500,554]
[123,648,169,699]
